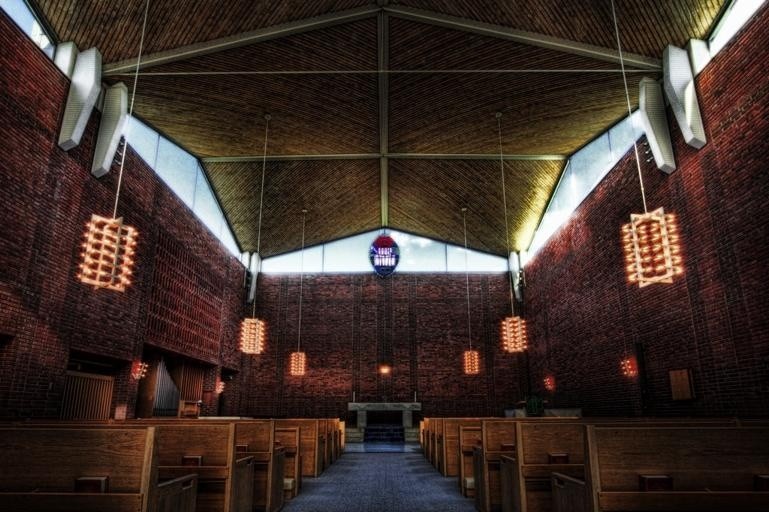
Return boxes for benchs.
[418,415,769,511]
[0,414,345,512]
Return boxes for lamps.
[461,206,480,375]
[75,1,151,295]
[494,112,528,353]
[289,209,309,376]
[239,114,273,356]
[610,0,687,290]
